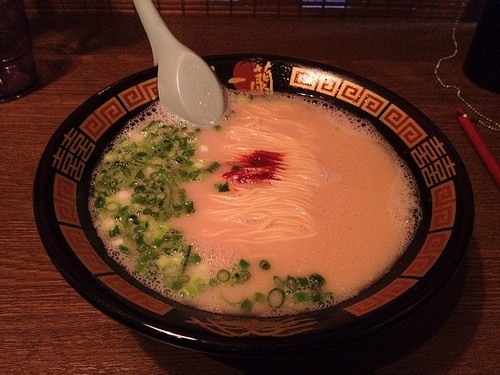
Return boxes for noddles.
[194,93,422,316]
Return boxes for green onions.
[90,116,336,311]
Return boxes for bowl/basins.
[32,54,474,358]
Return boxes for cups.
[0,33,41,102]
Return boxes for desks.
[1,15,500,374]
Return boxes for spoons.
[132,0,226,129]
